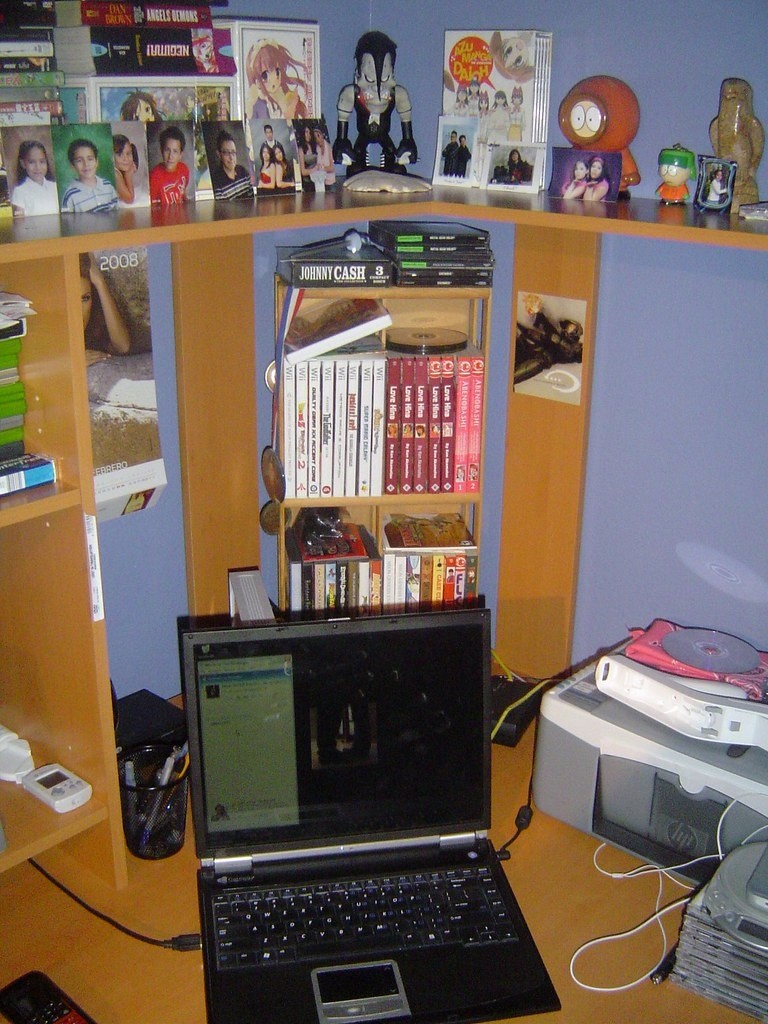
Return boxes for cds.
[662,629,761,673]
[388,326,467,345]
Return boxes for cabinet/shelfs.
[1,180,766,892]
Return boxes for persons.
[113,136,143,206]
[507,150,523,184]
[213,131,254,200]
[258,125,295,189]
[62,140,121,213]
[80,253,130,365]
[14,140,59,216]
[151,126,189,204]
[706,170,727,204]
[299,125,335,191]
[442,131,471,179]
[563,155,610,201]
[655,144,696,204]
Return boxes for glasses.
[220,150,237,157]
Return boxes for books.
[288,511,479,623]
[0,0,235,126]
[0,319,55,494]
[285,341,486,495]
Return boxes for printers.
[531,654,768,886]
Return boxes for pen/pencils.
[651,939,678,986]
[178,741,189,761]
[153,751,189,834]
[138,757,175,851]
[124,762,138,839]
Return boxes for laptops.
[180,607,563,1024]
